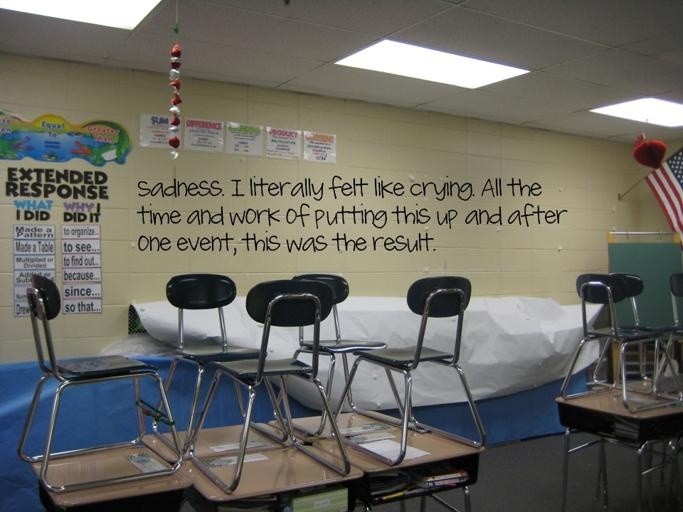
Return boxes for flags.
[645,150,683,249]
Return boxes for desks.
[554,388,682,512]
[593,378,682,511]
[32,444,196,512]
[140,420,308,468]
[180,441,365,512]
[268,409,402,443]
[310,426,482,510]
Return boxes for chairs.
[14,272,184,493]
[329,276,488,468]
[557,271,683,414]
[151,272,293,462]
[185,278,351,495]
[271,272,407,439]
